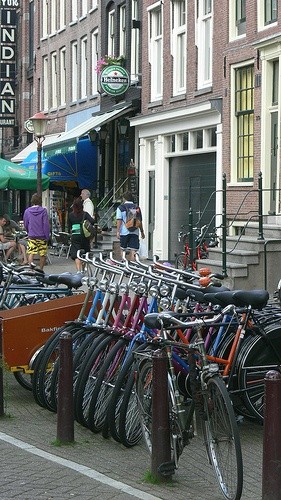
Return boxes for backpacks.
[119,205,142,232]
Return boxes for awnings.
[43,107,137,152]
[10,132,66,162]
[18,152,77,188]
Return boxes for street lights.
[27,110,53,208]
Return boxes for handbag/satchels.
[81,212,96,241]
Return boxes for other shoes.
[77,271,81,275]
[20,260,28,265]
[80,270,85,276]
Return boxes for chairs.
[0,232,72,264]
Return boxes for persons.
[116,191,145,262]
[66,202,98,274]
[23,193,51,272]
[81,189,94,249]
[0,214,29,265]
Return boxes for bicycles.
[0,223,281,500]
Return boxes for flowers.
[96,55,126,76]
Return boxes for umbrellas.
[0,158,50,218]
